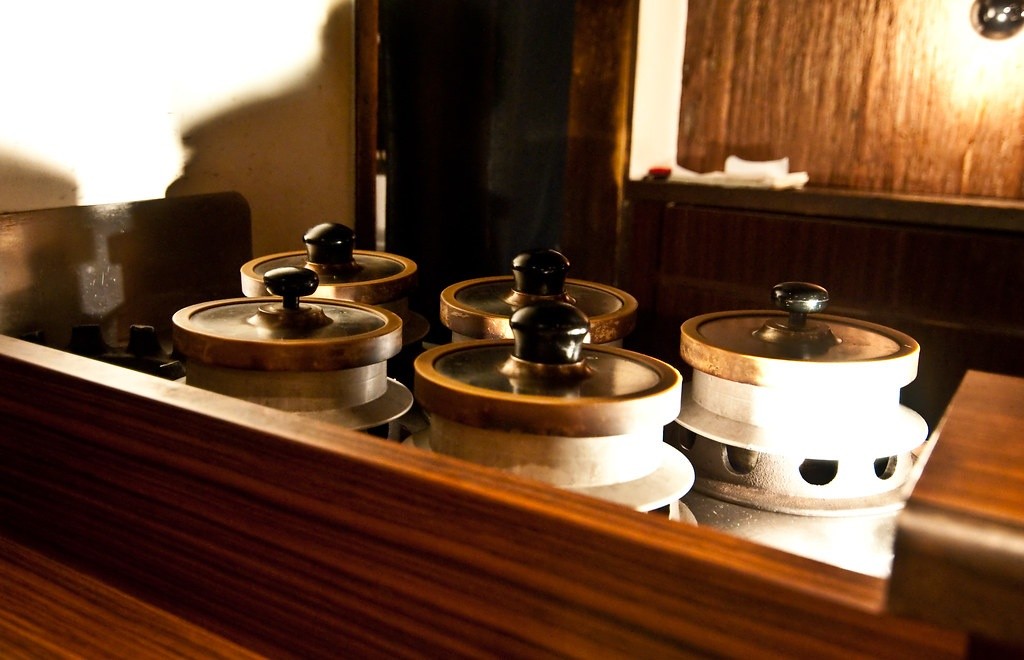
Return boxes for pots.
[170,223,928,535]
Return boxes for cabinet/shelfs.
[656,203,1023,439]
